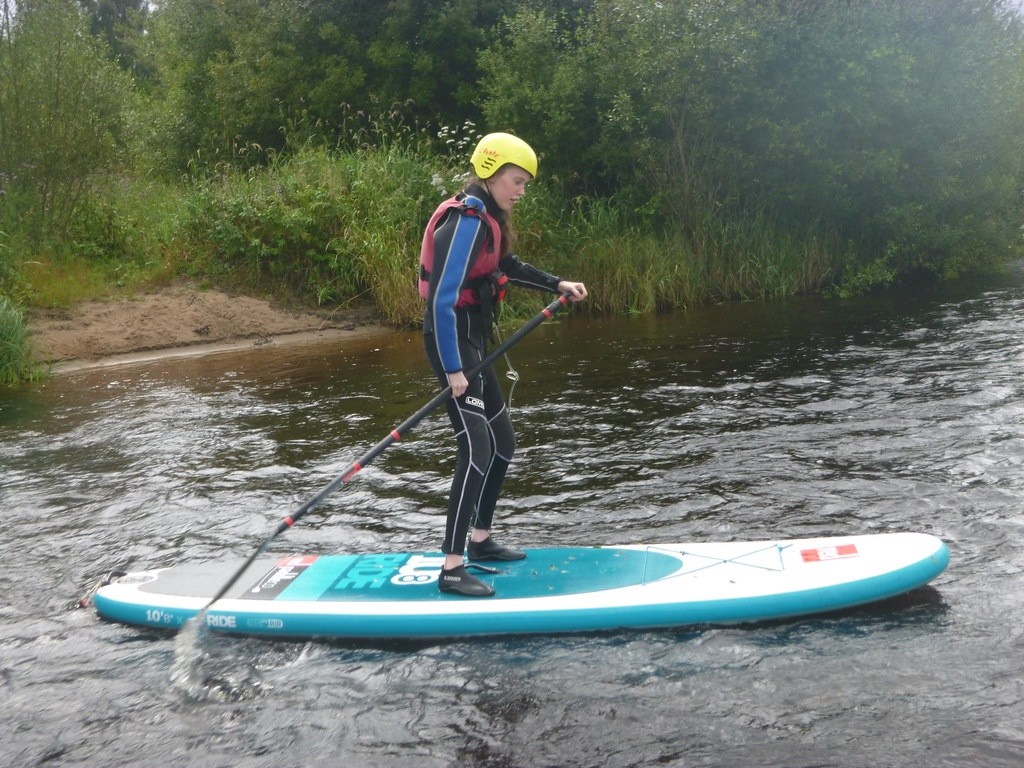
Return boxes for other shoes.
[467,533,526,560]
[438,564,496,596]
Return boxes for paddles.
[204,289,572,611]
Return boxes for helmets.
[469,132,538,180]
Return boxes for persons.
[420,133,588,596]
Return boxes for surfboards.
[95,532,949,641]
